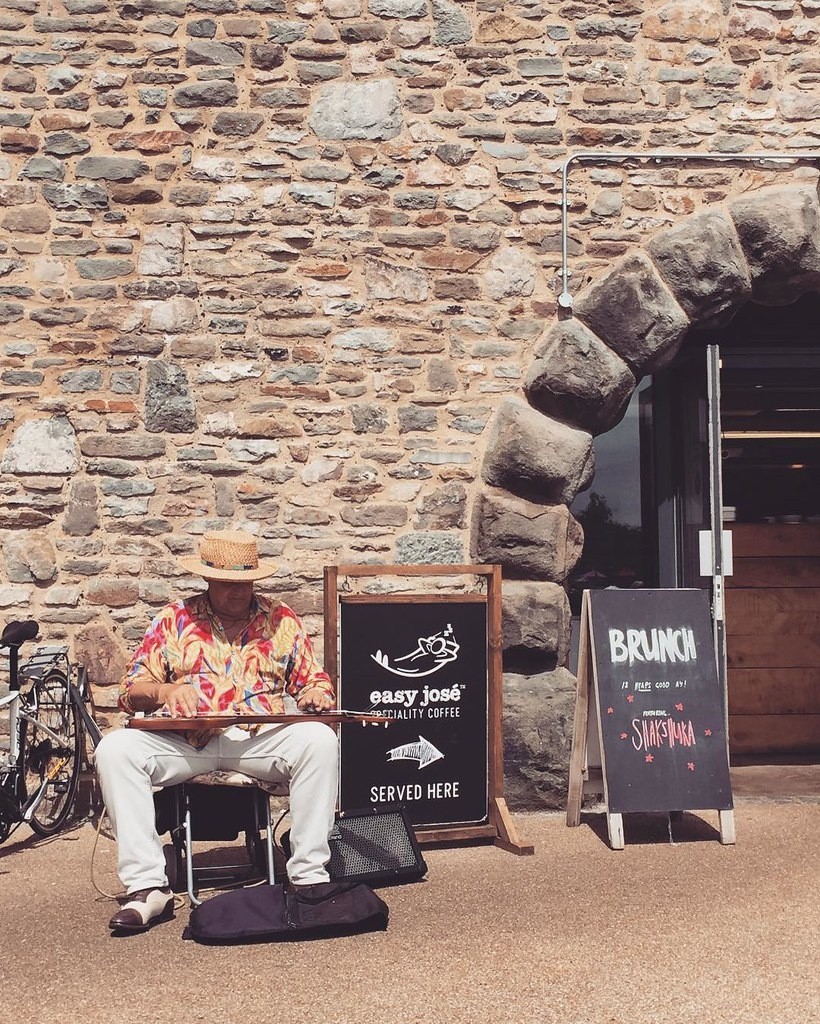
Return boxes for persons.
[88,529,342,935]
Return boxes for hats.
[180,531,279,582]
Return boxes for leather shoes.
[109,885,175,930]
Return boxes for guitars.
[125,708,397,731]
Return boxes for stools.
[173,769,276,907]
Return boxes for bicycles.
[0,621,85,845]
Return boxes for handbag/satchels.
[182,881,390,945]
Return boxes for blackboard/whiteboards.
[340,595,488,825]
[567,587,734,817]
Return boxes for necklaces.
[216,615,253,631]
